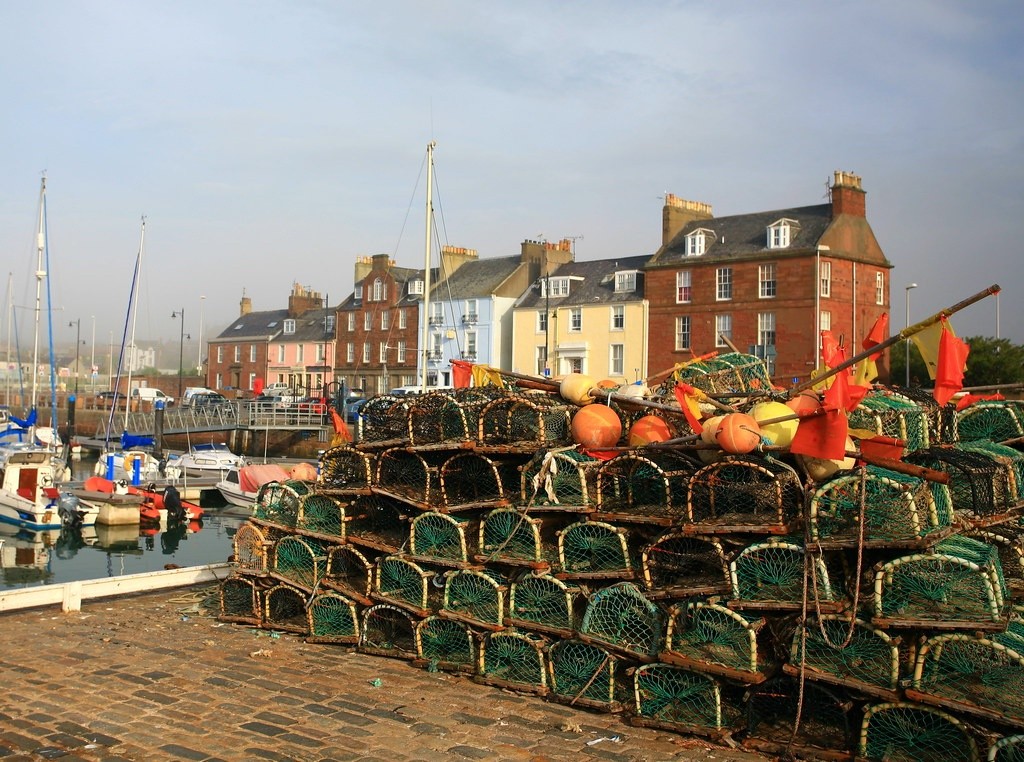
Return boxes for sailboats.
[0,167,247,531]
[0,521,63,589]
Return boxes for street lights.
[172,307,184,396]
[906,283,918,389]
[91,315,96,374]
[196,295,207,377]
[70,318,81,393]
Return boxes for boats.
[216,417,292,510]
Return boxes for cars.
[97,391,127,399]
[181,386,392,425]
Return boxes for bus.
[390,385,454,399]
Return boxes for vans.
[133,387,175,406]
